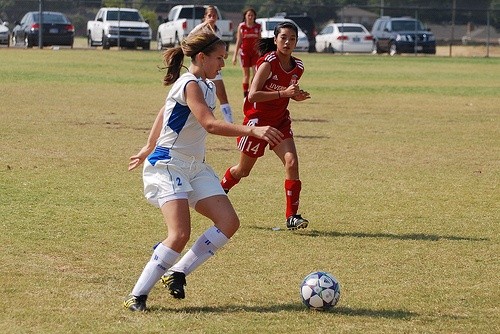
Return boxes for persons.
[219,21,310,230]
[231,8,262,102]
[197,4,233,124]
[122,28,287,310]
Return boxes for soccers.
[300,271,340,310]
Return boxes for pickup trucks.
[86,7,153,49]
[157,4,234,51]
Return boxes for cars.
[256,17,309,52]
[11,10,75,48]
[0,19,11,47]
[315,23,374,54]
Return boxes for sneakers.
[286,214,309,231]
[123,294,151,313]
[160,270,187,299]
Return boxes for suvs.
[272,11,317,47]
[372,16,437,55]
